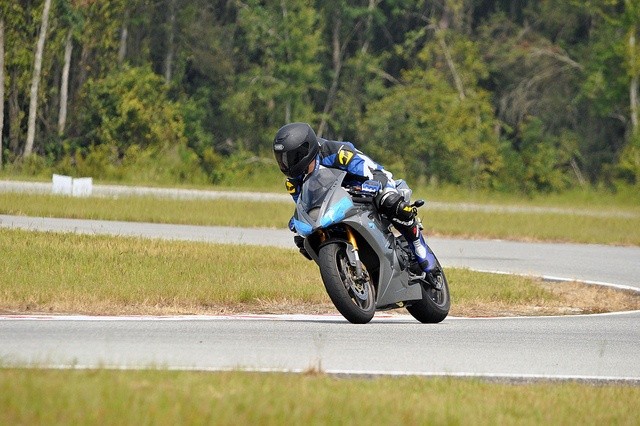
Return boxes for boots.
[408,224,436,273]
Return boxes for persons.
[272,123,436,273]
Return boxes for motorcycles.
[293,167,451,324]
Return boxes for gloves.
[361,180,382,198]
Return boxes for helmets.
[274,122,321,179]
